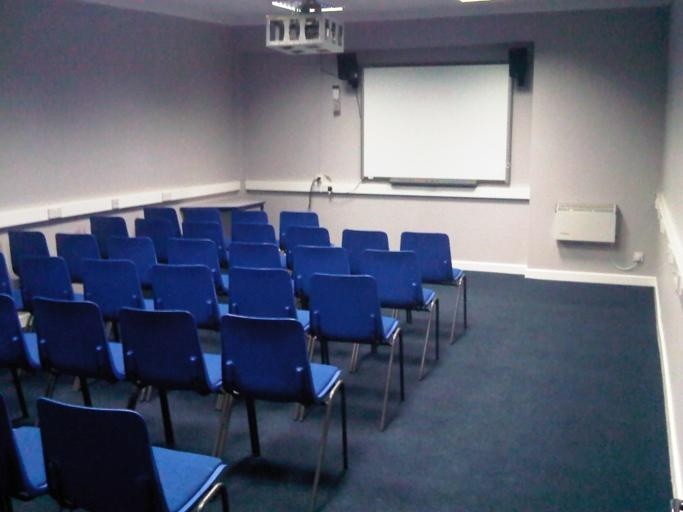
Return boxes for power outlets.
[630,249,645,264]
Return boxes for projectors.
[266,13,344,54]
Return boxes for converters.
[634,253,641,261]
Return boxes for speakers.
[509,47,527,77]
[338,54,356,81]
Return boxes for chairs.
[299,273,404,430]
[36,397,229,511]
[352,249,440,381]
[1,400,49,510]
[228,211,390,357]
[1,205,229,450]
[213,314,348,511]
[393,230,468,345]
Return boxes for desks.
[178,200,264,237]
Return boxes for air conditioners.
[553,199,618,246]
